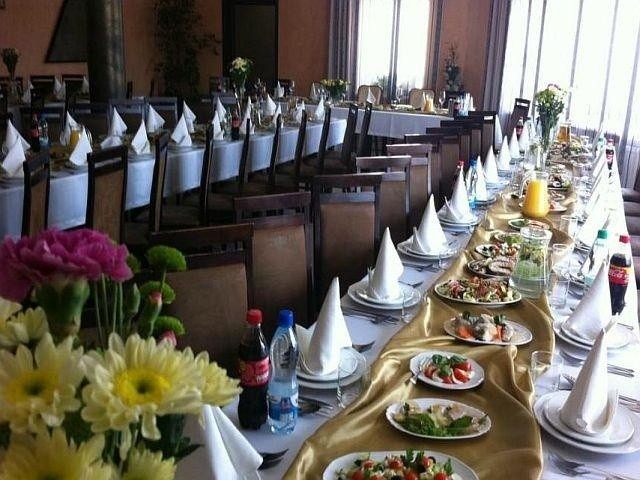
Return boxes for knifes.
[342,308,400,323]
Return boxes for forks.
[559,349,635,378]
[352,341,375,353]
[340,305,391,323]
[298,395,333,421]
[397,280,423,289]
[547,448,634,479]
[402,262,433,272]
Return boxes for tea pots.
[519,170,551,218]
[67,122,92,155]
[510,227,552,299]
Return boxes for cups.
[531,152,593,404]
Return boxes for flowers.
[0,224,243,480]
[534,83,570,154]
[229,57,255,97]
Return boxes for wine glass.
[285,80,447,118]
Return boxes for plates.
[296,139,640,479]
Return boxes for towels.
[175,404,268,480]
[558,148,628,439]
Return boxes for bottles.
[594,138,615,174]
[29,113,50,152]
[243,311,269,425]
[589,225,610,293]
[609,235,630,310]
[453,95,462,118]
[225,107,239,142]
[266,306,302,437]
